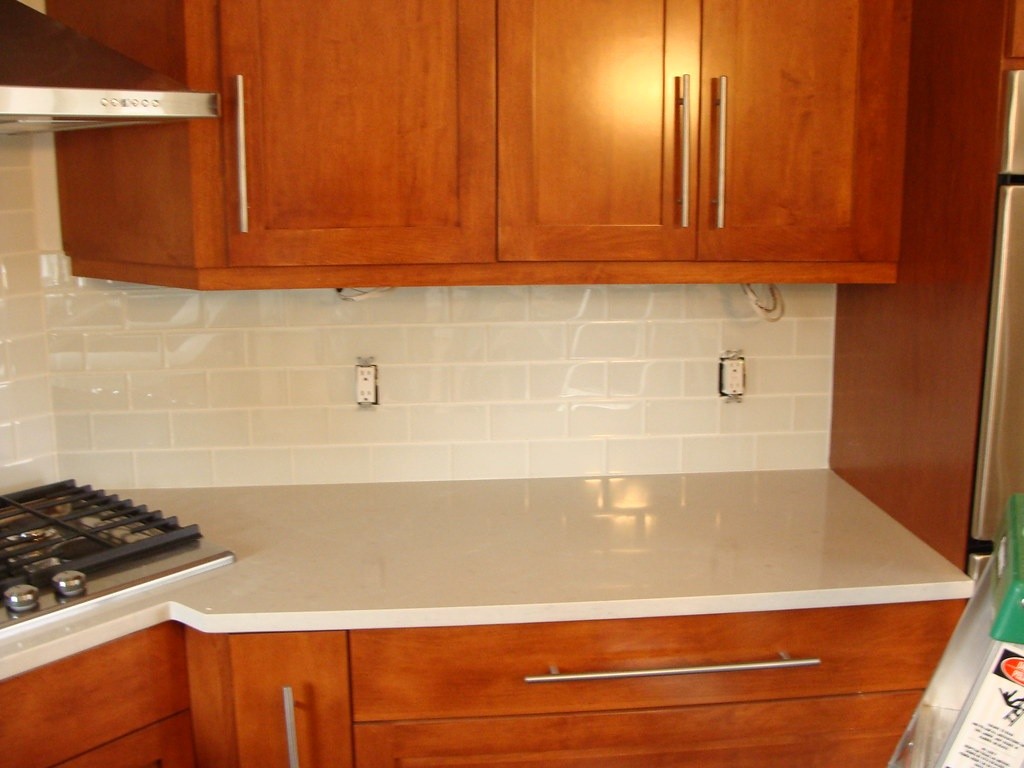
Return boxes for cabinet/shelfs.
[500,1,913,269]
[48,0,497,270]
[0,621,195,767]
[346,598,969,768]
[185,630,353,768]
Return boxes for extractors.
[0,0,223,135]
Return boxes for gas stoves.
[0,479,236,640]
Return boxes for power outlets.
[721,359,743,395]
[356,365,376,404]
